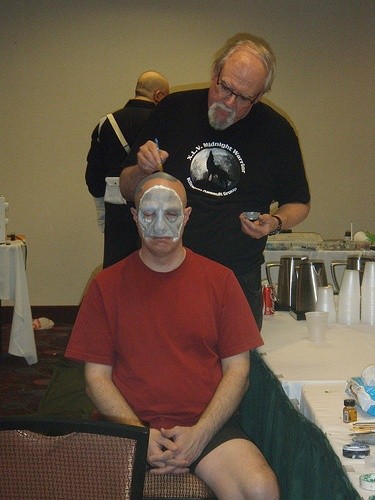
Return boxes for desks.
[0,239,39,368]
[260,249,375,292]
[296,384,375,500]
[231,294,375,410]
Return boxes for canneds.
[262,284,275,316]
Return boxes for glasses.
[216,65,262,105]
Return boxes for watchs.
[268,216,282,237]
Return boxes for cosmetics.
[342,443,370,459]
[359,473,375,491]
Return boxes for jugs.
[331,257,375,294]
[265,255,329,316]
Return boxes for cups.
[314,261,375,326]
[305,311,329,343]
[243,212,260,225]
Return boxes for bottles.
[344,232,351,240]
[343,398,357,423]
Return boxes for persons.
[121,41,311,332]
[85,71,169,271]
[64,171,280,500]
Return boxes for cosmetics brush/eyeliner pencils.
[156,138,163,172]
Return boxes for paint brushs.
[154,137,163,173]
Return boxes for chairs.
[0,414,150,500]
[142,463,214,500]
